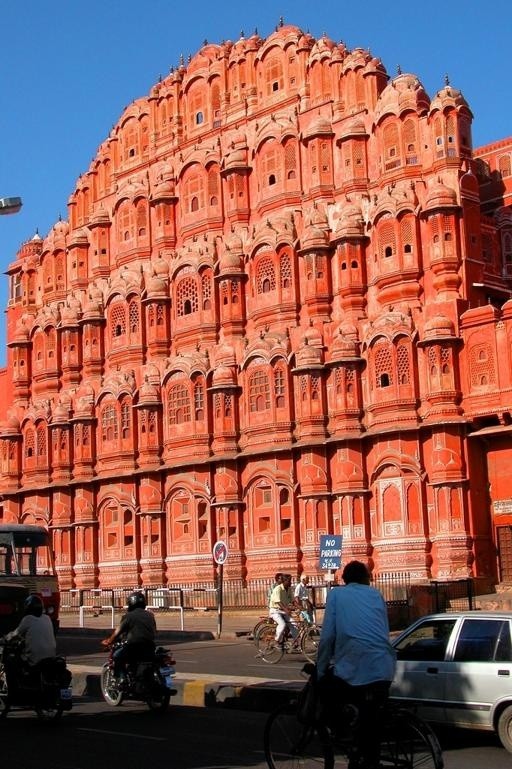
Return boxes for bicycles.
[252,603,324,671]
[262,658,454,767]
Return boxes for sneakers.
[273,640,283,650]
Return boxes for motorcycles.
[99,636,177,716]
[0,631,72,722]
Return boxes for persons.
[313,558,397,768]
[294,573,315,625]
[3,594,59,676]
[267,572,294,615]
[101,592,157,687]
[269,573,300,651]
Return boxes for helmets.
[24,595,43,617]
[127,592,146,612]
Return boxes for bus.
[0,522,61,639]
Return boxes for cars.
[368,608,512,757]
